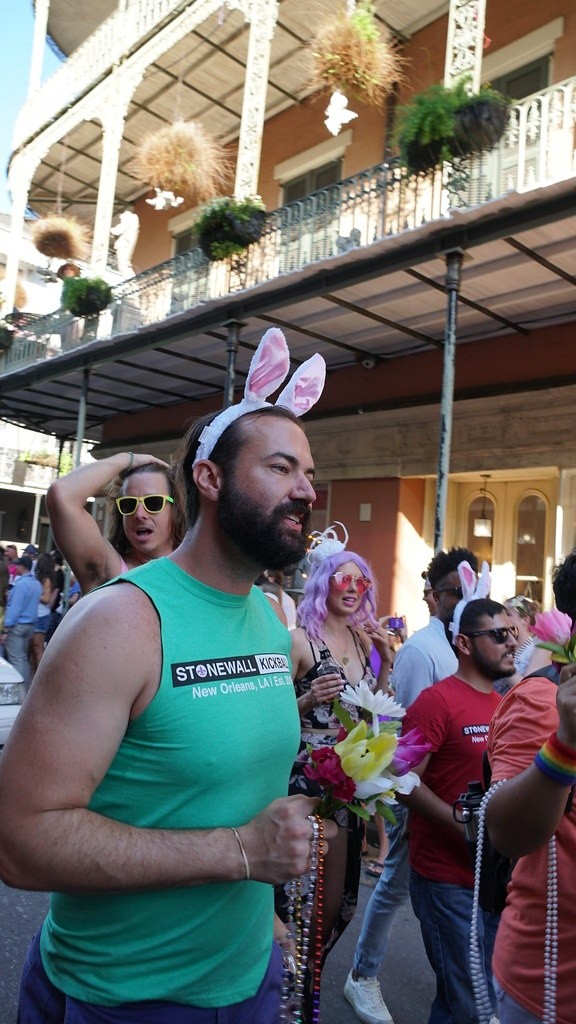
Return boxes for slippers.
[367,859,385,877]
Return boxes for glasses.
[115,492,176,515]
[331,571,373,594]
[433,584,464,600]
[470,627,520,646]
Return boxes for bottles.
[316,645,344,703]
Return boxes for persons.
[401,599,518,1024]
[491,594,552,700]
[289,523,392,965]
[48,452,180,597]
[1,545,82,687]
[112,211,140,284]
[0,404,337,1024]
[362,614,406,875]
[489,552,576,1024]
[341,549,478,1024]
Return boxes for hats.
[22,545,38,555]
[11,556,31,567]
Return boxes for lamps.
[474,473,492,539]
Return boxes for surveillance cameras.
[363,358,376,369]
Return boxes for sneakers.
[343,966,394,1024]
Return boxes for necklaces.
[513,637,533,661]
[471,778,557,1024]
[326,632,349,665]
[280,816,324,1023]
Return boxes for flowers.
[192,192,266,226]
[34,212,90,259]
[302,678,435,825]
[131,126,227,199]
[308,5,404,101]
[524,609,575,667]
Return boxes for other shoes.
[362,848,369,855]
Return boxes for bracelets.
[119,452,134,478]
[534,732,576,786]
[231,827,249,879]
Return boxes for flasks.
[454,782,510,878]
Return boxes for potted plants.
[387,83,503,173]
[61,277,113,317]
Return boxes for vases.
[201,213,267,260]
